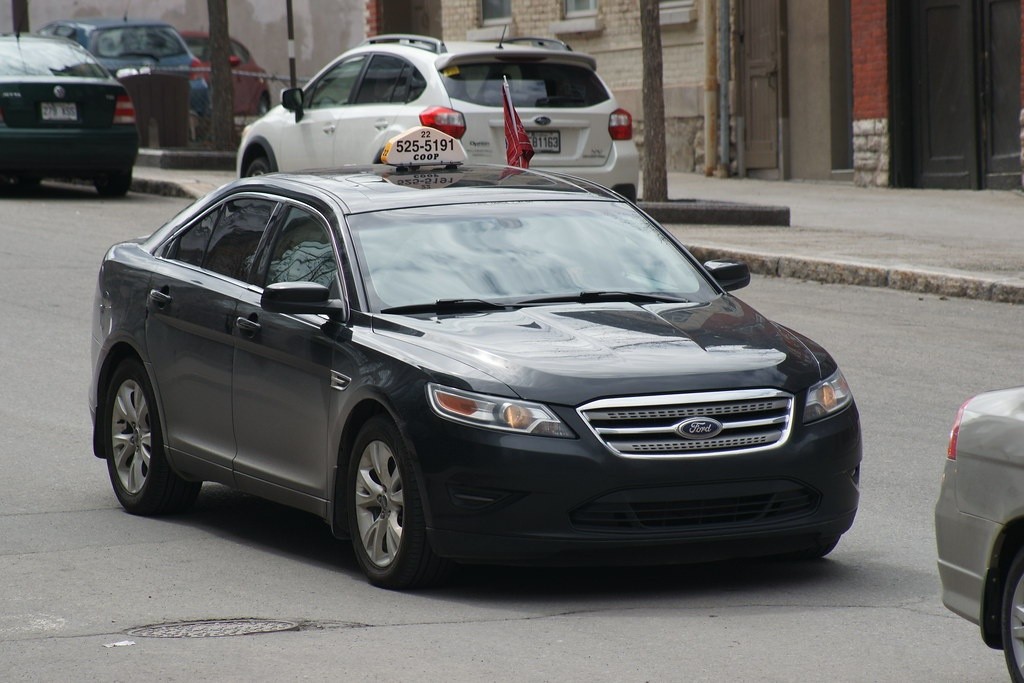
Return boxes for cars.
[82,125,865,594]
[35,17,211,148]
[0,29,142,198]
[176,29,272,123]
[934,385,1024,683]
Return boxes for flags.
[502,86,535,170]
[496,167,523,182]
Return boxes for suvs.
[228,29,642,213]
